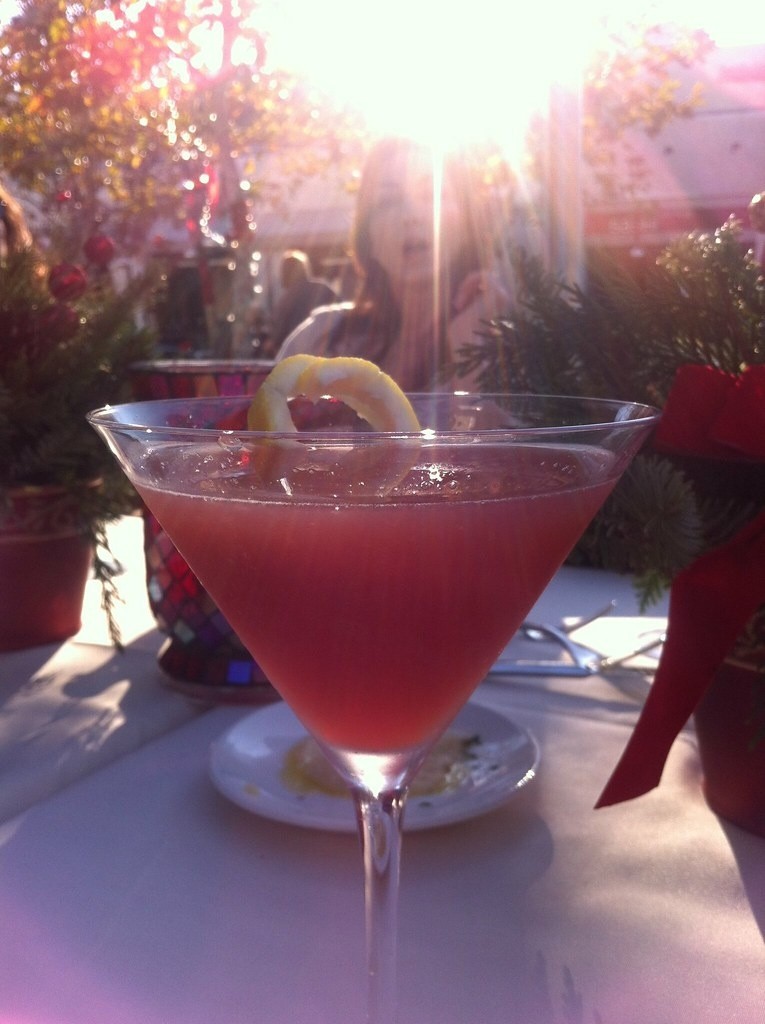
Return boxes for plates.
[209,696,540,832]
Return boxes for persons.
[261,251,337,372]
[270,132,534,427]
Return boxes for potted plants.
[644,215,765,837]
[0,254,170,655]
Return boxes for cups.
[127,359,360,704]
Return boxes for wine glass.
[85,392,662,1024]
